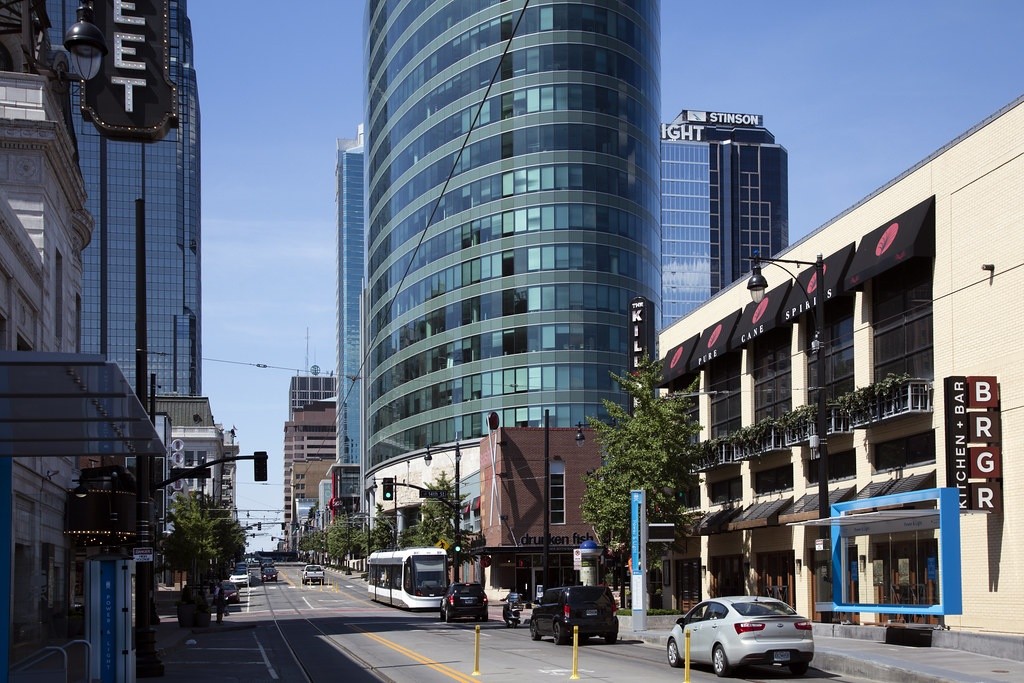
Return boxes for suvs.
[529,585,620,645]
[440,582,489,622]
[259,556,275,568]
[415,579,444,597]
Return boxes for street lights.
[740,248,834,623]
[137,373,269,677]
[424,443,462,582]
[372,476,398,549]
[316,508,331,568]
[573,417,617,448]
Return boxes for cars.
[666,596,815,677]
[608,586,631,609]
[235,562,250,573]
[260,567,279,582]
[244,557,260,567]
[214,581,241,604]
[228,569,252,587]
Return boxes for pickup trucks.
[301,565,325,586]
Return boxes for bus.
[368,547,450,612]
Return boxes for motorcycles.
[500,593,523,628]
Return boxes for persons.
[209,579,215,594]
[500,587,521,619]
[214,582,225,624]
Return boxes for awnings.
[843,195,935,291]
[730,277,792,350]
[781,241,856,323]
[688,471,935,537]
[689,307,742,370]
[654,332,700,389]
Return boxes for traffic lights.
[382,477,394,500]
